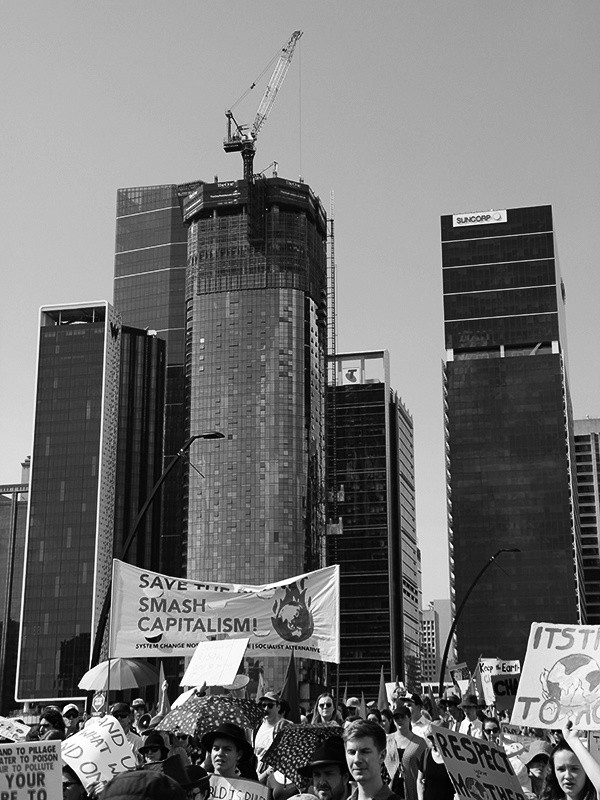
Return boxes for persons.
[0,682,600,800]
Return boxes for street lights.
[85,429,225,718]
[437,546,521,698]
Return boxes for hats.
[259,691,281,705]
[201,722,254,763]
[297,735,346,777]
[138,734,170,761]
[110,698,147,715]
[345,697,360,707]
[61,704,80,717]
[456,695,481,709]
[439,695,460,706]
[97,769,186,800]
[391,691,424,718]
[521,740,554,765]
[179,764,214,789]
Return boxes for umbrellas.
[153,695,266,741]
[78,657,160,691]
[263,723,391,794]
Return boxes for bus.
[420,682,455,701]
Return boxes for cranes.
[222,29,304,181]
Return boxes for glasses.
[319,703,332,708]
[261,703,277,709]
[142,747,161,754]
[63,712,79,719]
[63,782,78,792]
[369,718,378,723]
[380,720,386,722]
[38,724,50,729]
[113,713,130,719]
[176,734,189,741]
[188,791,201,800]
[483,727,500,735]
